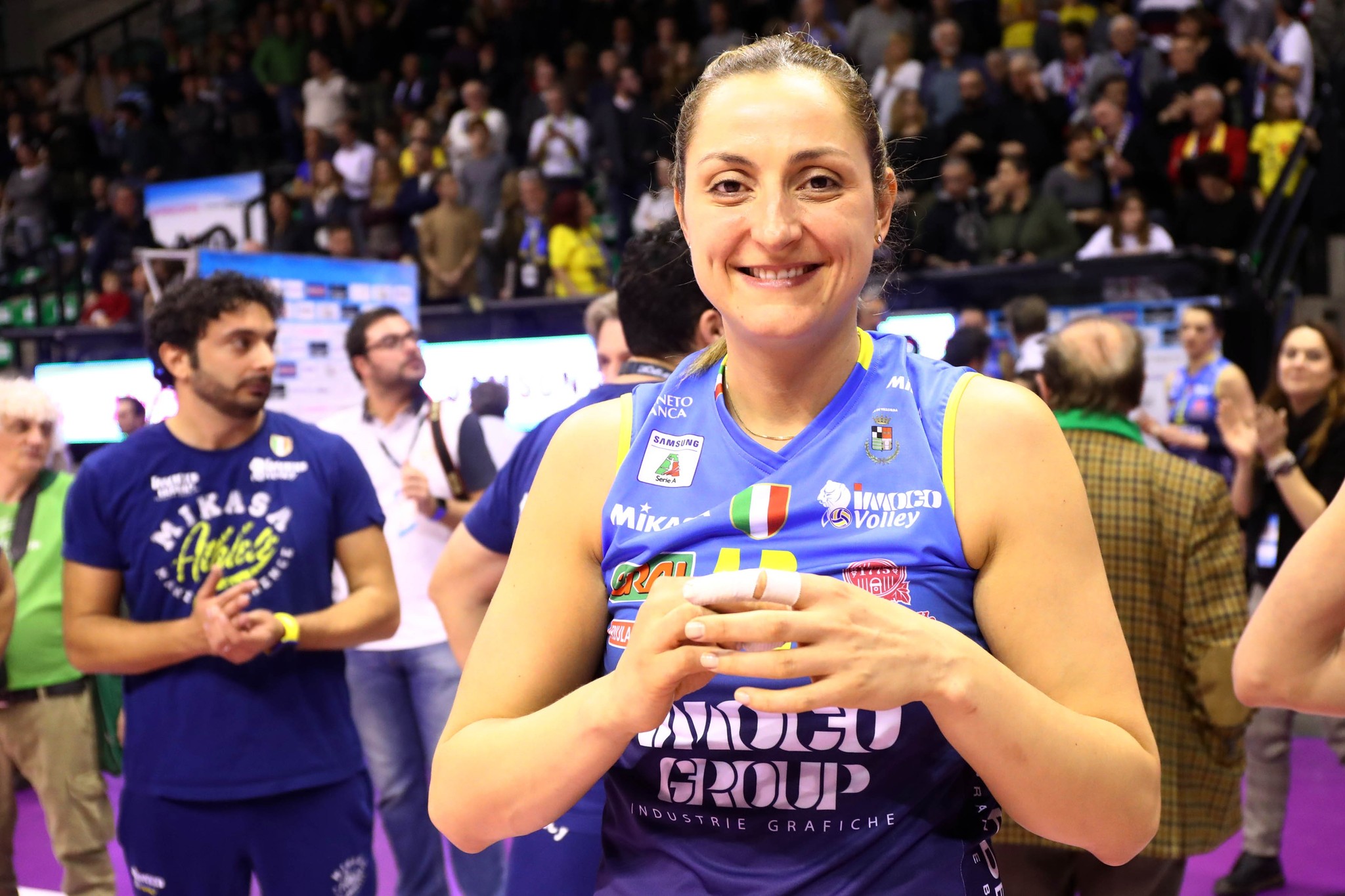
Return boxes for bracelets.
[1266,450,1298,475]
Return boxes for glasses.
[366,329,418,350]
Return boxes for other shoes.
[1213,852,1285,895]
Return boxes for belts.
[6,677,87,704]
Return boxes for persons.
[1,0,1345,896]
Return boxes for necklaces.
[723,368,794,441]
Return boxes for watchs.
[275,610,300,649]
[429,496,447,521]
